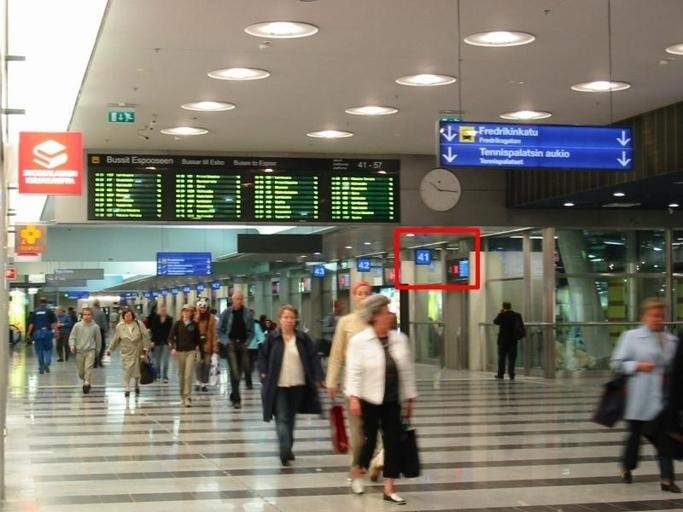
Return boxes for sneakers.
[494,374,515,380]
[82,384,91,394]
[154,378,169,383]
[124,388,139,397]
[92,364,103,368]
[619,469,633,483]
[660,481,682,493]
[39,366,50,374]
[180,399,192,407]
[229,396,241,408]
[279,450,295,466]
[193,385,208,392]
[56,358,68,362]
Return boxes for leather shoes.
[383,492,407,506]
[350,478,365,495]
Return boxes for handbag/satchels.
[396,424,422,479]
[139,352,156,384]
[194,360,210,384]
[595,375,627,430]
[330,404,349,456]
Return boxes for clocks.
[417,167,461,212]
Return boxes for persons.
[27,296,58,374]
[325,281,385,482]
[110,307,144,330]
[144,300,220,408]
[493,301,526,381]
[257,304,324,465]
[106,310,151,396]
[661,333,682,443]
[69,307,102,393]
[610,297,680,493]
[343,294,416,505]
[55,299,109,368]
[245,308,277,389]
[217,291,255,409]
